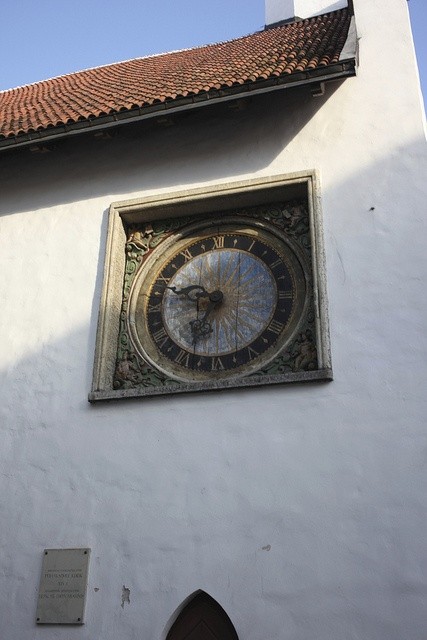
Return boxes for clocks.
[87,170,334,400]
